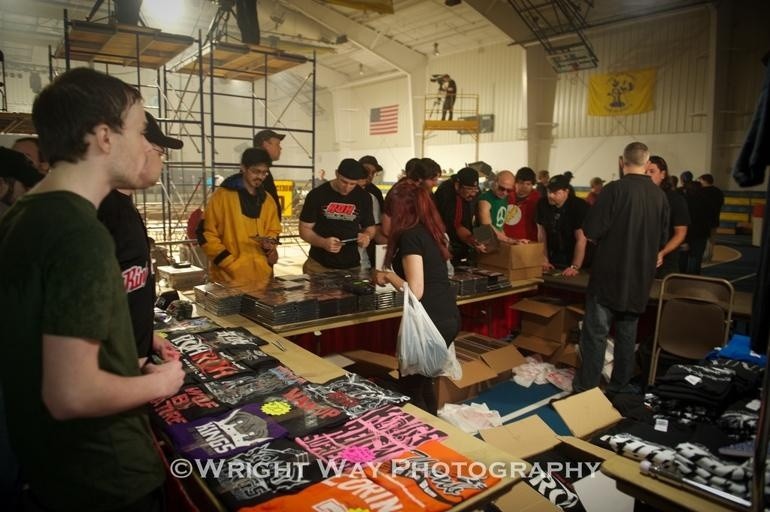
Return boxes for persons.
[0,66,186,512]
[240,130,285,223]
[299,128,725,276]
[442,74,456,122]
[0,146,46,222]
[571,143,671,403]
[235,1,260,45]
[196,148,282,287]
[373,178,462,416]
[12,137,50,173]
[97,110,184,372]
[115,0,143,26]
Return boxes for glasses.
[154,149,169,161]
[497,184,513,193]
[462,187,479,192]
[249,168,270,176]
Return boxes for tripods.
[428,82,443,120]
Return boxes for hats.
[254,130,286,145]
[144,111,183,149]
[338,159,363,180]
[545,175,568,193]
[450,167,478,187]
[359,156,383,171]
[0,146,46,186]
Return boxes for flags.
[369,104,399,136]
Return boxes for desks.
[141,285,539,512]
[601,453,738,512]
[545,271,753,315]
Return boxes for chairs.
[648,274,735,385]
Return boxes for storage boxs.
[475,242,545,282]
[324,296,625,512]
